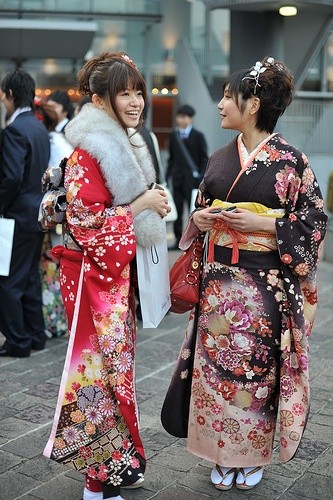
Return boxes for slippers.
[214,464,236,490]
[235,466,262,489]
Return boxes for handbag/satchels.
[0,215,14,276]
[169,231,203,313]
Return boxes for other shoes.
[167,244,179,251]
[0,345,10,357]
[120,476,144,489]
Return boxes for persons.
[38,52,171,500]
[0,70,209,358]
[162,55,329,490]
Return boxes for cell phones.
[210,206,237,215]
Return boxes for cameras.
[149,183,164,192]
